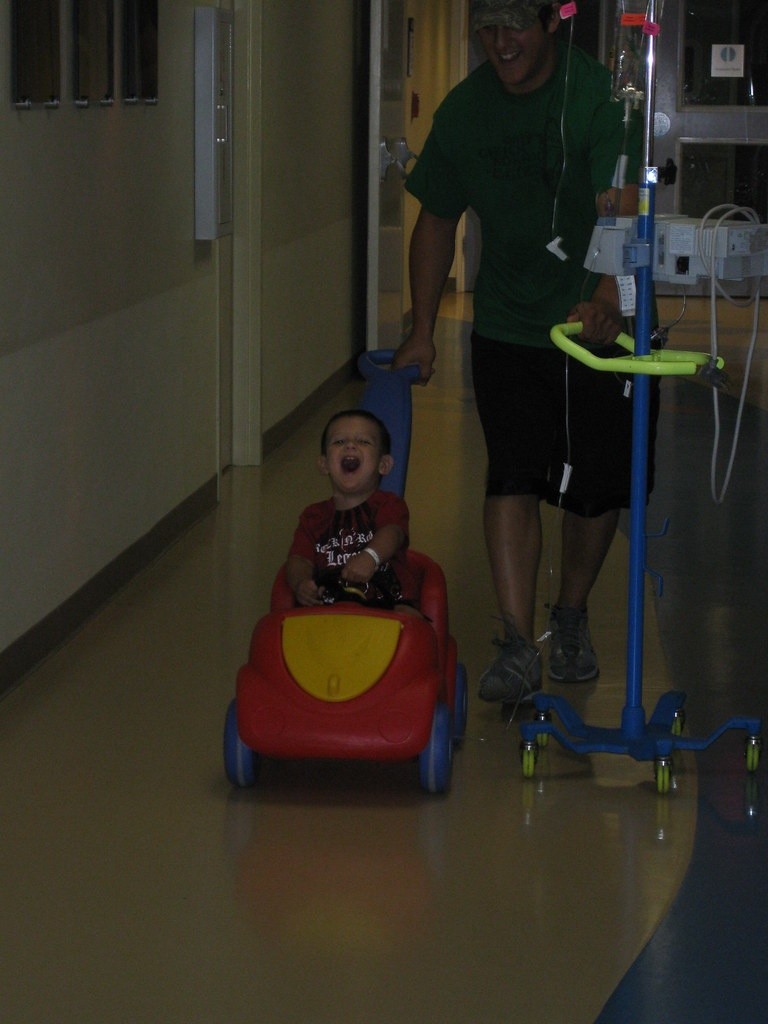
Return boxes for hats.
[470,0,557,32]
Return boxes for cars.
[223,546,469,795]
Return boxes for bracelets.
[363,547,380,570]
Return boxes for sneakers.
[477,633,543,702]
[545,603,600,682]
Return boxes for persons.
[284,409,410,607]
[391,0,662,705]
